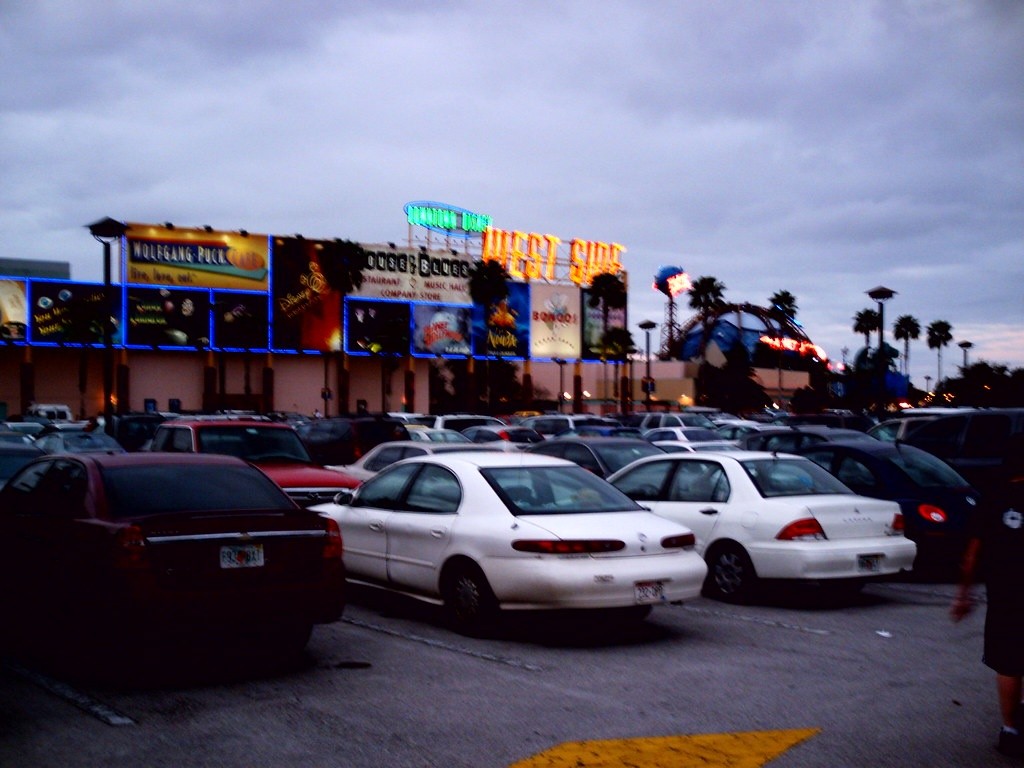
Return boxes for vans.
[899,408,1024,494]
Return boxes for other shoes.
[998,727,1024,759]
[1017,703,1024,729]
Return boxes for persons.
[348,304,384,353]
[131,290,209,345]
[223,295,267,347]
[312,409,321,419]
[948,436,1024,759]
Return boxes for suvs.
[296,416,411,468]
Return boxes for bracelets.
[952,599,972,606]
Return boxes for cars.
[390,410,883,477]
[305,453,709,637]
[0,448,343,675]
[799,442,981,581]
[1,402,165,485]
[593,451,917,602]
[146,416,363,510]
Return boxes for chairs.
[502,486,542,507]
